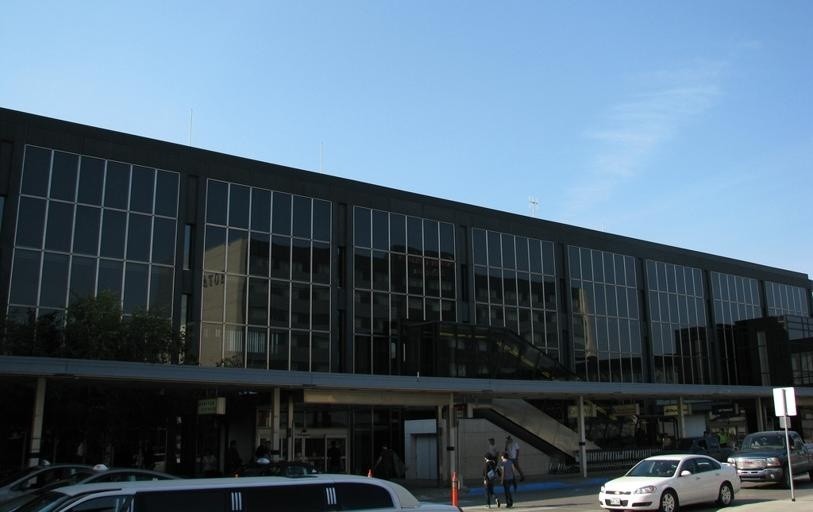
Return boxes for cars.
[598,454,741,512]
[0,464,462,511]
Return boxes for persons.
[478,433,525,507]
[130,435,343,475]
[373,447,400,480]
[658,418,731,454]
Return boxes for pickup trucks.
[728,430,813,487]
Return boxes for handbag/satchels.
[487,469,496,480]
